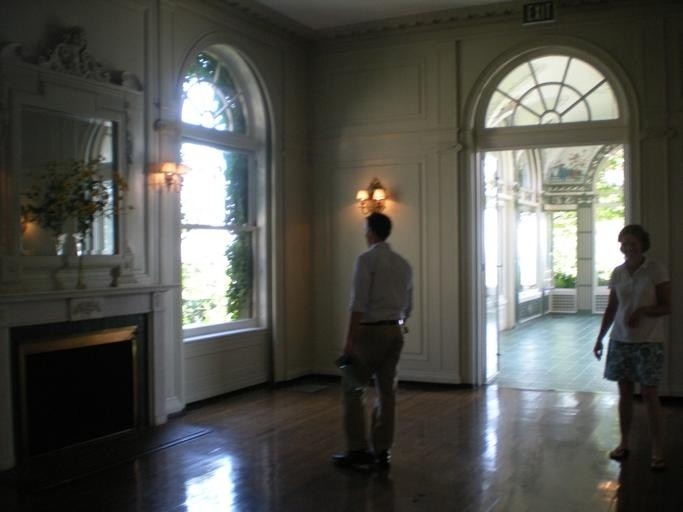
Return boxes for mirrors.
[1,25,144,291]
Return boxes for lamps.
[160,161,192,192]
[356,177,390,215]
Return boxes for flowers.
[18,154,136,291]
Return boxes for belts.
[357,319,405,327]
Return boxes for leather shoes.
[374,449,393,470]
[330,448,372,473]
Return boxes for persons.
[328,210,415,471]
[592,223,674,473]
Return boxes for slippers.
[609,447,630,460]
[649,454,666,472]
